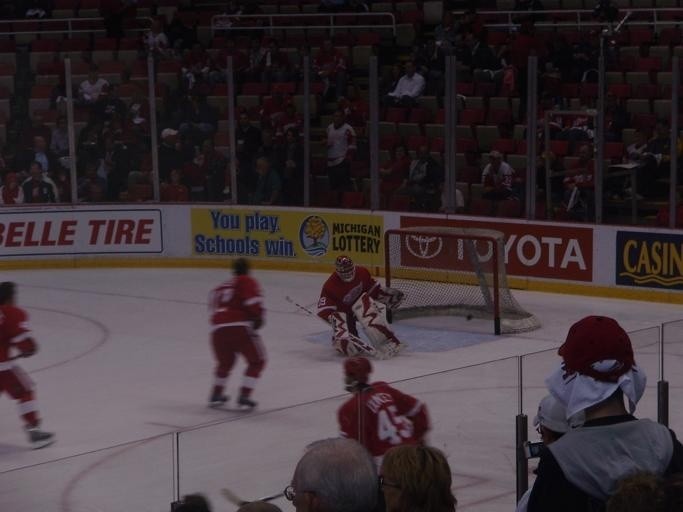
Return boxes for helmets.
[335,255,356,282]
[343,357,372,385]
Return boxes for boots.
[24,419,55,442]
[208,394,231,404]
[237,396,259,407]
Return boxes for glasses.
[284,484,313,502]
[378,476,400,491]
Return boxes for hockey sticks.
[221,487,283,507]
[286,296,406,360]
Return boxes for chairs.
[1,0,682,229]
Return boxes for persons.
[0,280,57,443]
[291,437,379,512]
[316,254,406,360]
[205,257,267,407]
[513,313,683,511]
[221,488,283,512]
[0,1,682,232]
[175,492,210,512]
[379,444,458,512]
[337,357,432,472]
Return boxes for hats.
[486,150,503,160]
[159,127,179,139]
[558,315,635,378]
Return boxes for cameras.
[523,441,544,459]
